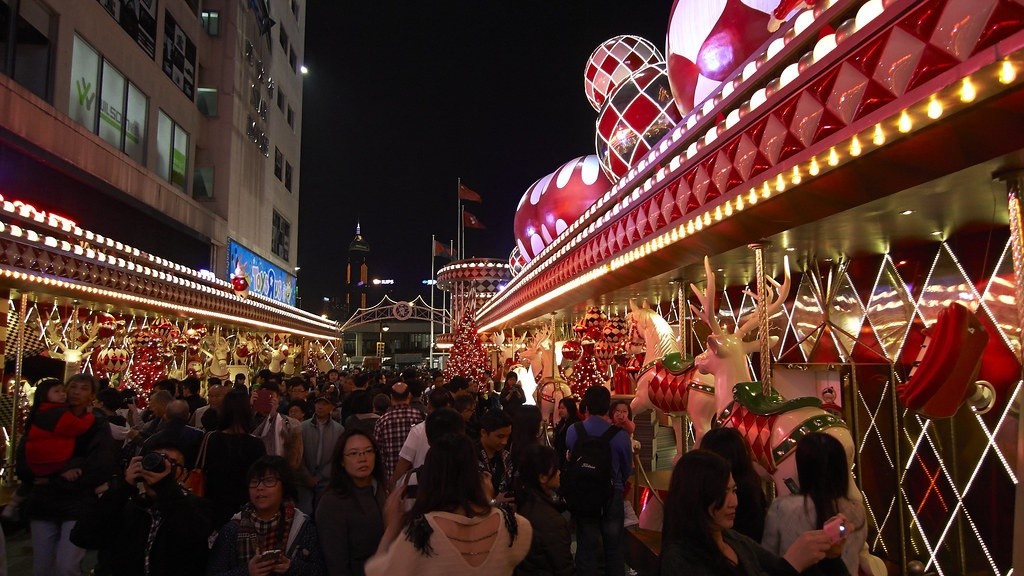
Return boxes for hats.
[314,394,333,404]
[427,388,462,413]
[136,425,206,472]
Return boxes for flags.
[460,184,481,202]
[434,240,457,258]
[465,211,485,228]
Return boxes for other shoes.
[2,502,21,522]
[622,500,640,527]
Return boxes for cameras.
[135,453,177,482]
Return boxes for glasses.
[248,476,282,487]
[464,408,476,414]
[343,449,376,458]
[291,390,307,394]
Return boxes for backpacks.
[569,421,622,520]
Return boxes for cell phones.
[784,478,799,495]
[823,518,845,545]
[402,485,418,499]
[262,550,281,565]
[256,390,274,402]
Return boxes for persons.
[15,368,867,576]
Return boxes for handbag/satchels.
[185,431,213,497]
